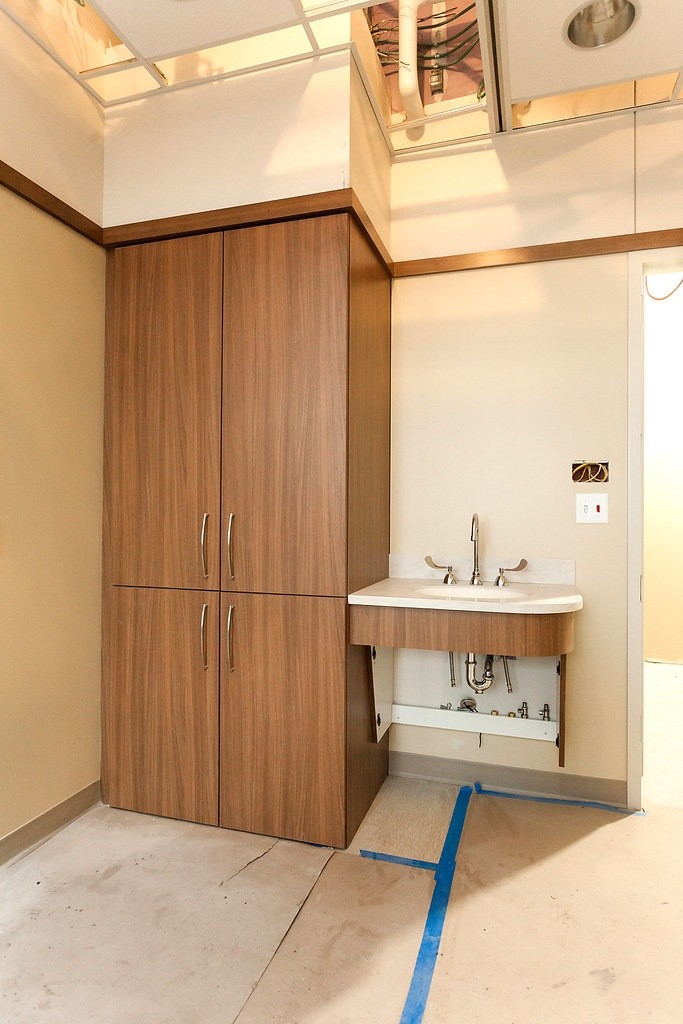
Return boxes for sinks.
[415,586,534,599]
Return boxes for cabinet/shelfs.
[101,213,390,850]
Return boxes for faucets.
[470,513,483,586]
